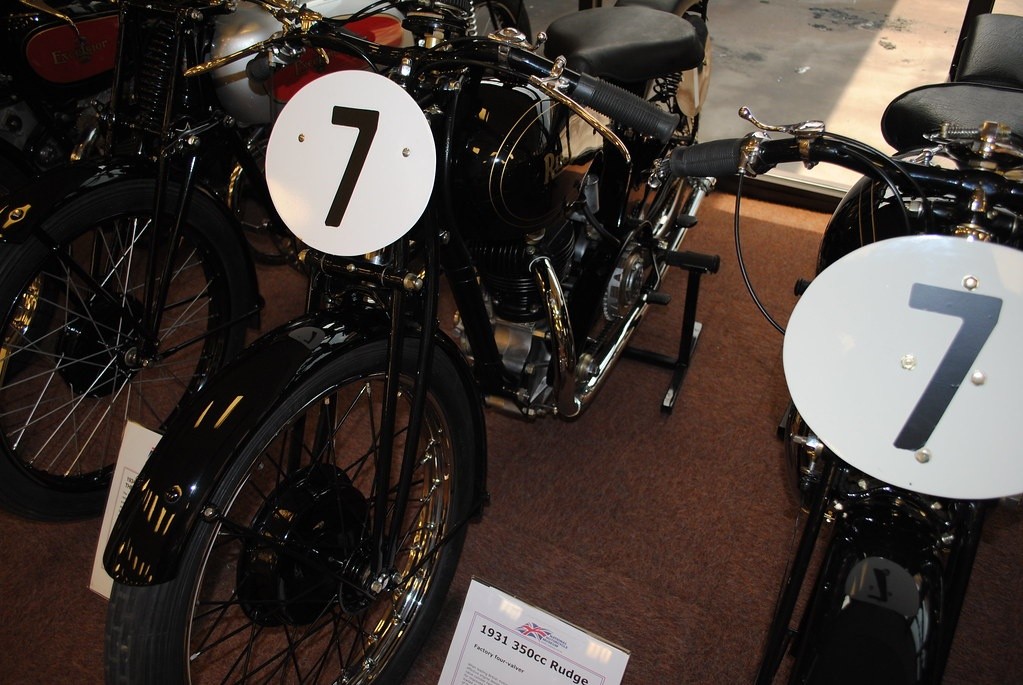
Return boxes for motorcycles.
[666,14,1023,685]
[0,0,722,685]
